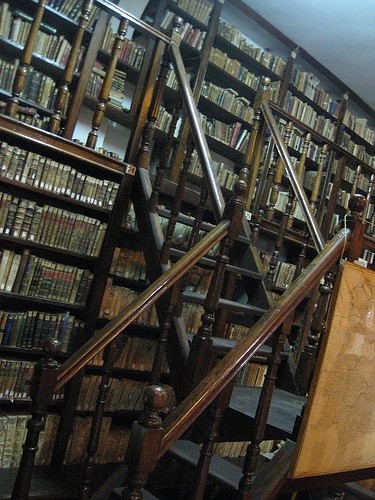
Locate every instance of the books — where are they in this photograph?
[0,0,375,500]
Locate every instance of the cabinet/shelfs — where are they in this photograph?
[0,0,375,500]
